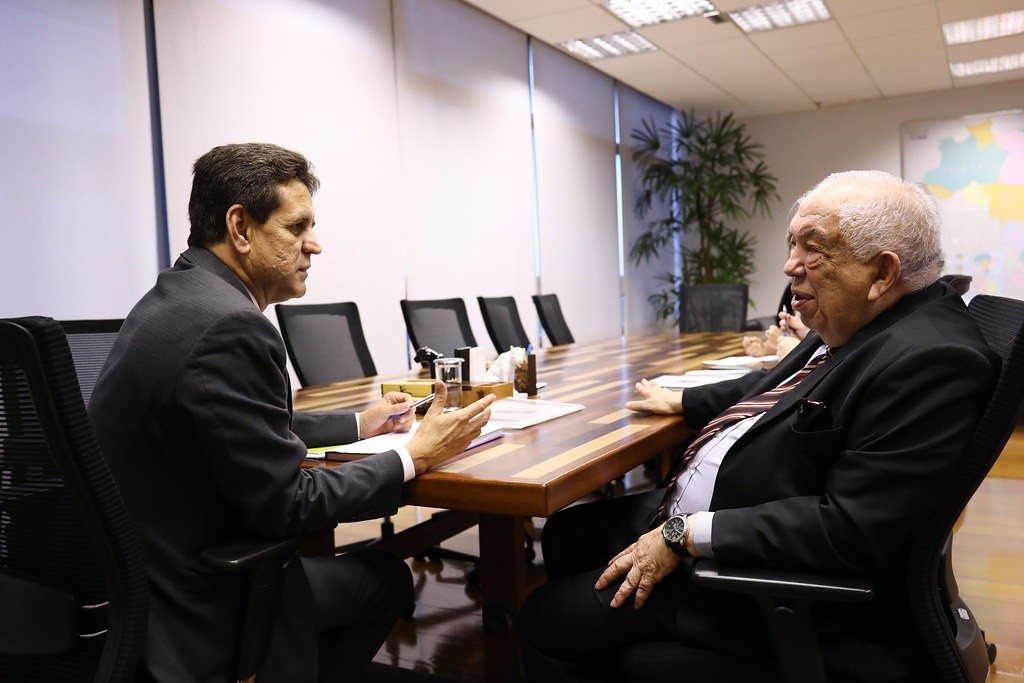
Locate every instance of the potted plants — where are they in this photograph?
[621,110,786,332]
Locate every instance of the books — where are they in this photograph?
[304,421,505,461]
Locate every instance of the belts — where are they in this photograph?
[647,510,693,574]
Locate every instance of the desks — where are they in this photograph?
[290,333,804,683]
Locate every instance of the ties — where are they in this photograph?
[646,346,837,531]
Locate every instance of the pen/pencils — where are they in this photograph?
[389,393,436,418]
[782,303,788,340]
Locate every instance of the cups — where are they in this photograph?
[433,357,462,414]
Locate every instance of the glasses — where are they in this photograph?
[797,397,834,432]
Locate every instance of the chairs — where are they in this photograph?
[944,275,973,295]
[275,301,379,389]
[532,293,573,345]
[478,296,531,354]
[0,314,404,683]
[59,319,126,410]
[400,297,478,370]
[586,295,1024,683]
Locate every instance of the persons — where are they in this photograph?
[69,143,497,683]
[517,170,1004,683]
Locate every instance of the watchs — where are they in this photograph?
[661,513,693,558]
